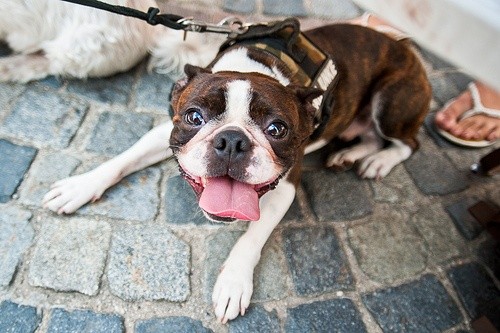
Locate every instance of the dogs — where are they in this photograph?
[40,22,435,324]
[0,0,205,84]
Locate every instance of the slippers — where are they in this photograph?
[434,79,500,147]
[361,11,408,41]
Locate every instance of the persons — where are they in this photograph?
[348,12,500,148]
